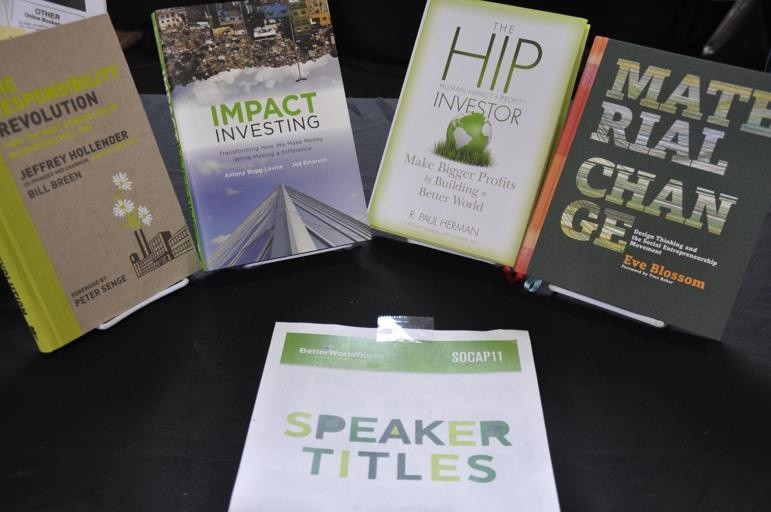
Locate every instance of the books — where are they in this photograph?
[365,0,591,267]
[0,0,106,41]
[147,0,377,273]
[512,35,770,346]
[230,323,566,512]
[0,11,204,356]
